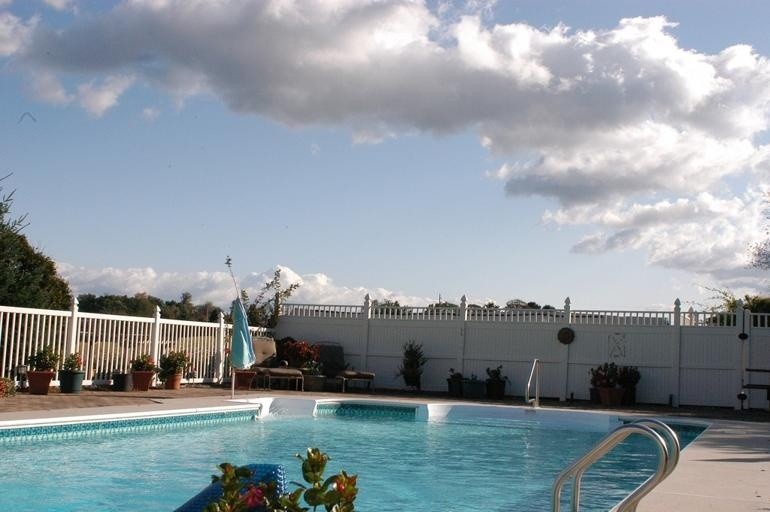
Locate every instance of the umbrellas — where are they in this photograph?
[229,297,256,398]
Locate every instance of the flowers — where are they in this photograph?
[64,351,85,368]
[132,354,156,371]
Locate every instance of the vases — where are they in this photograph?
[235,370,256,390]
[112,372,132,390]
[132,371,157,391]
[58,369,86,394]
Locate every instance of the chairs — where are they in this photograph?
[317,340,377,393]
[247,335,303,392]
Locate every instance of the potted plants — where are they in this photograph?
[586,362,640,408]
[394,340,429,391]
[302,341,326,393]
[22,345,59,394]
[159,349,192,390]
[446,363,511,400]
